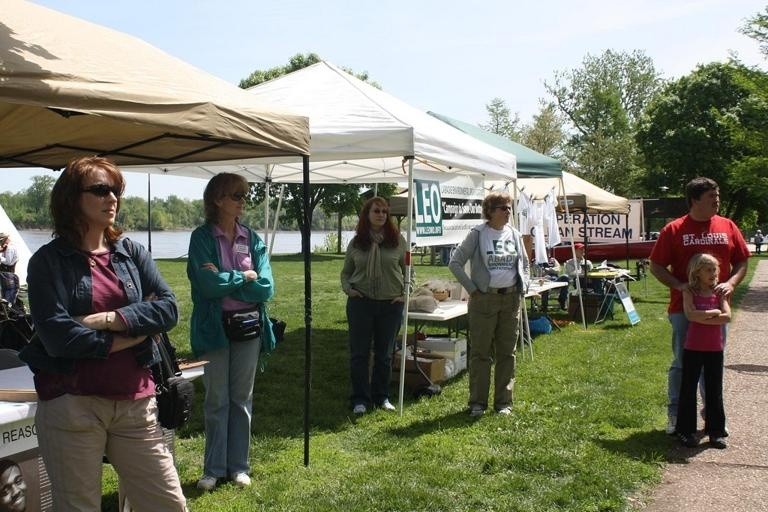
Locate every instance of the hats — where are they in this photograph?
[574,243,584,250]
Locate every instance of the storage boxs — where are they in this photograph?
[567,292,601,322]
[391,350,447,390]
[417,335,468,374]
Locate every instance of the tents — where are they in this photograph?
[0,204,32,284]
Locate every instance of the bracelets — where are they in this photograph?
[704,309,706,318]
[105,311,112,330]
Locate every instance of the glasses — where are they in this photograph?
[81,183,124,197]
[223,191,248,202]
[365,207,388,216]
[495,206,511,212]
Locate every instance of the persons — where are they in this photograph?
[17,155,187,512]
[449,192,530,417]
[649,177,752,437]
[340,196,414,415]
[531,245,568,311]
[187,173,276,491]
[676,253,731,448]
[0,460,27,512]
[754,230,763,254]
[0,232,19,301]
[564,243,592,288]
[0,233,19,273]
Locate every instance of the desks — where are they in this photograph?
[0,360,207,511]
[577,268,629,324]
[405,274,565,393]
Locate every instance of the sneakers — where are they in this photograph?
[353,404,366,414]
[231,469,252,487]
[381,400,395,411]
[197,473,217,490]
[667,409,729,449]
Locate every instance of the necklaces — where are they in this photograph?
[85,252,98,265]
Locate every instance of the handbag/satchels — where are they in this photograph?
[222,308,262,341]
[154,378,195,430]
